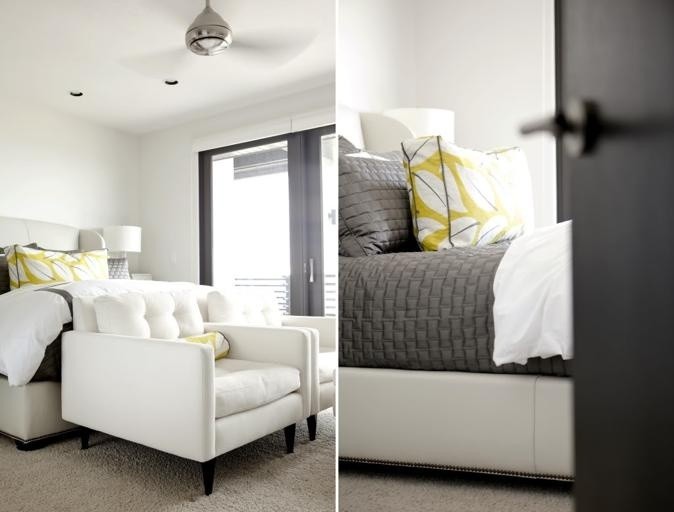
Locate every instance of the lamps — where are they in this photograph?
[107,226,143,259]
[184,1,229,57]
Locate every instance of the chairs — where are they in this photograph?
[59,288,335,496]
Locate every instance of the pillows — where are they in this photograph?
[5,244,108,291]
[337,135,525,258]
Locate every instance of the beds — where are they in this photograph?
[337,141,572,484]
[0,239,220,449]
